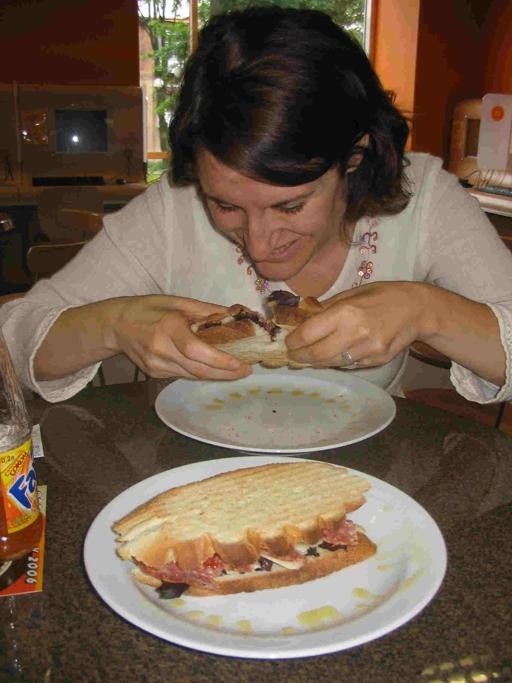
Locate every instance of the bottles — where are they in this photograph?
[0,327,44,563]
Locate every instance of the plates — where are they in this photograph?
[154,368,396,456]
[81,457,448,662]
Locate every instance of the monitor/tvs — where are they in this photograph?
[14,84,143,182]
[447,99,481,187]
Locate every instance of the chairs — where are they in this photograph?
[0,202,151,388]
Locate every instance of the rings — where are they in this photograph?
[342,351,355,363]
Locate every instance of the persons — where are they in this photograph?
[1,4,512,411]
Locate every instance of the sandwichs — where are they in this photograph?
[189,290,326,371]
[110,461,376,601]
